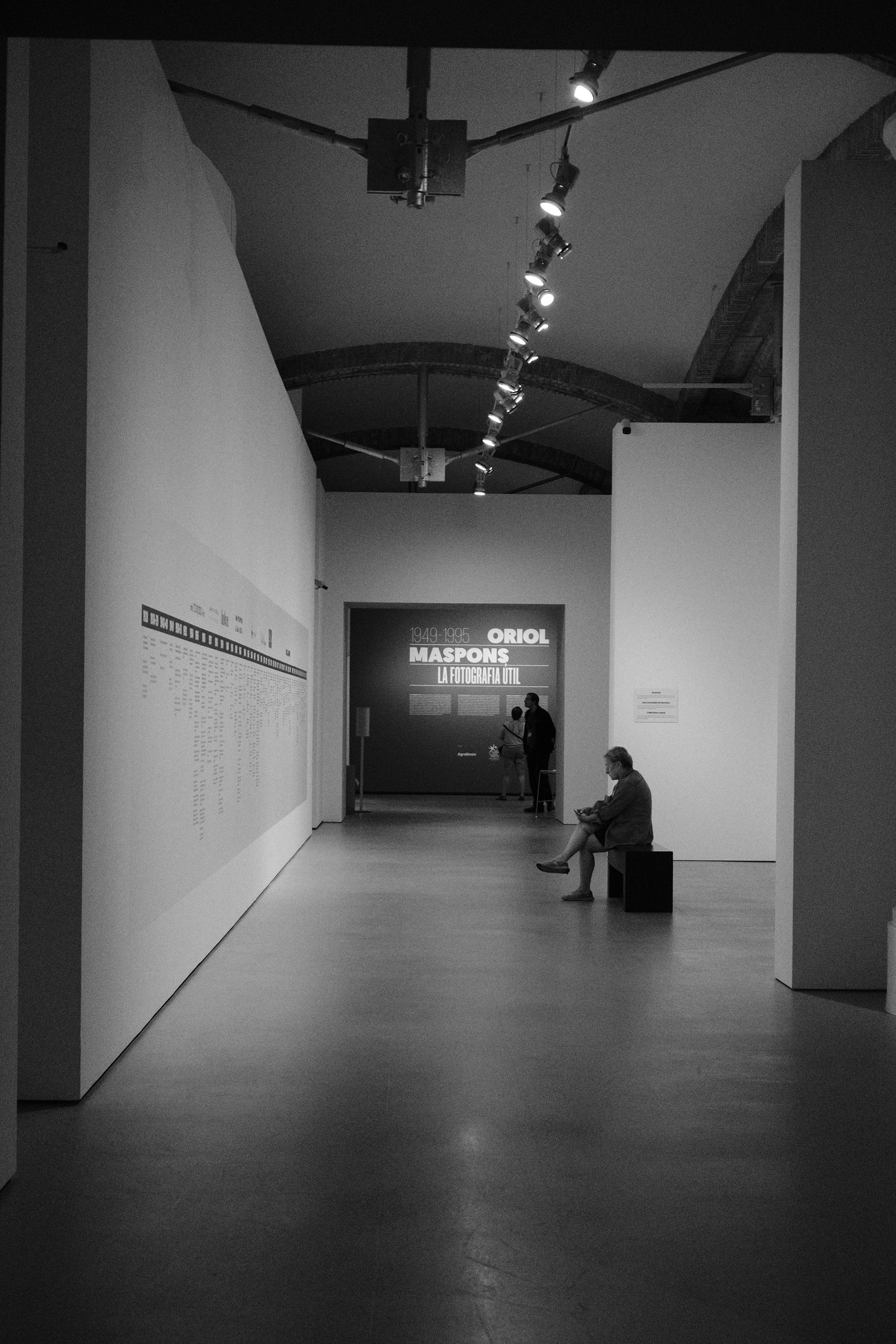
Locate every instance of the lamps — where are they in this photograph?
[356,706,371,813]
[473,47,619,496]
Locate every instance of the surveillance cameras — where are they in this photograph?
[621,419,632,434]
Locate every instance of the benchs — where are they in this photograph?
[607,840,674,913]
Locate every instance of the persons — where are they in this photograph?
[522,692,557,813]
[536,747,654,901]
[494,706,527,801]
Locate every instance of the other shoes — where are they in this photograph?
[524,801,545,812]
[496,795,507,800]
[518,797,524,800]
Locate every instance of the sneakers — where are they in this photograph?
[536,859,570,874]
[561,888,595,901]
